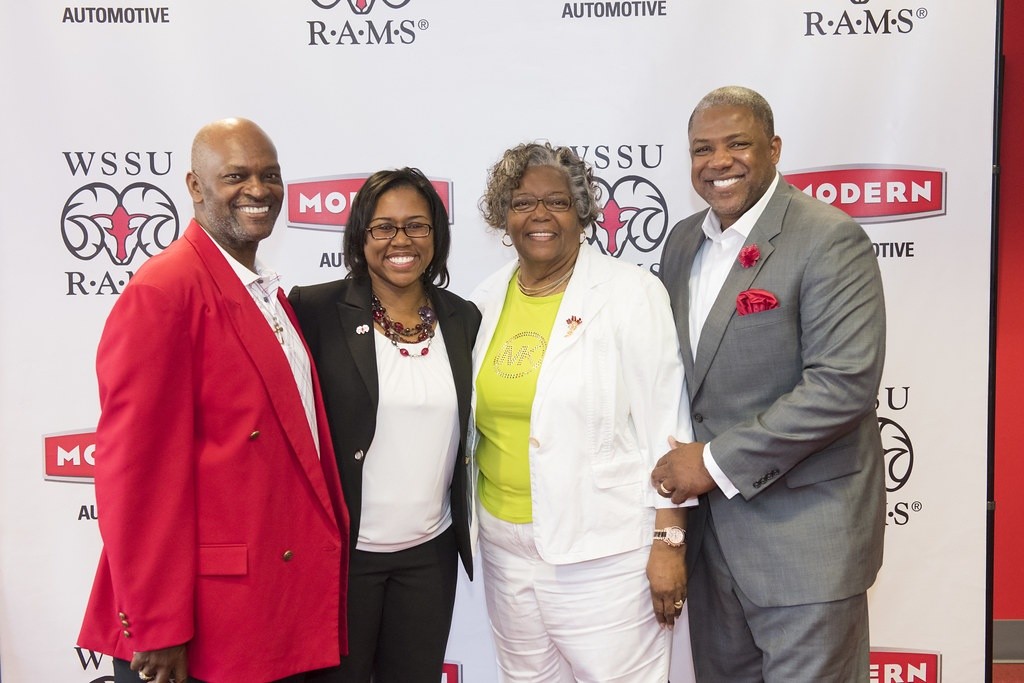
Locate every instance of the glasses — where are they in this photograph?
[366,223,434,240]
[510,191,576,213]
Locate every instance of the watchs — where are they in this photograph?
[653,526,687,548]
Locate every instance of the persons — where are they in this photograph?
[651,84,887,683]
[77,118,350,683]
[288,167,483,683]
[467,143,699,683]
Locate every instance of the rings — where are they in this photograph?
[139,671,152,681]
[674,600,683,609]
[660,484,672,494]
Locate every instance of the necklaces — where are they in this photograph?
[371,294,436,357]
[517,268,574,297]
[251,290,283,343]
